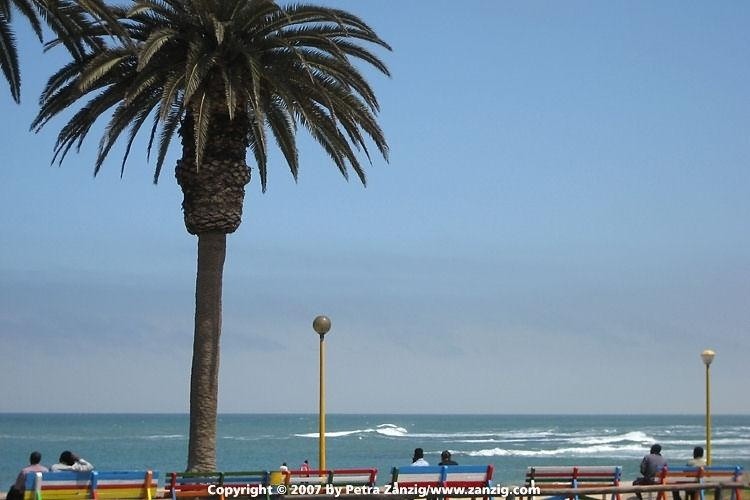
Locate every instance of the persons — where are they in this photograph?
[280,462,288,471]
[300,460,312,469]
[672,446,706,500]
[5,451,49,500]
[438,450,457,465]
[51,450,95,472]
[632,443,667,500]
[410,448,428,466]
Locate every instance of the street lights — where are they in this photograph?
[701,350,715,466]
[313,316,331,477]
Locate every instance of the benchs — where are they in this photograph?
[165,471,268,500]
[23,472,159,500]
[269,470,378,500]
[649,465,744,500]
[519,466,623,500]
[388,464,494,500]
